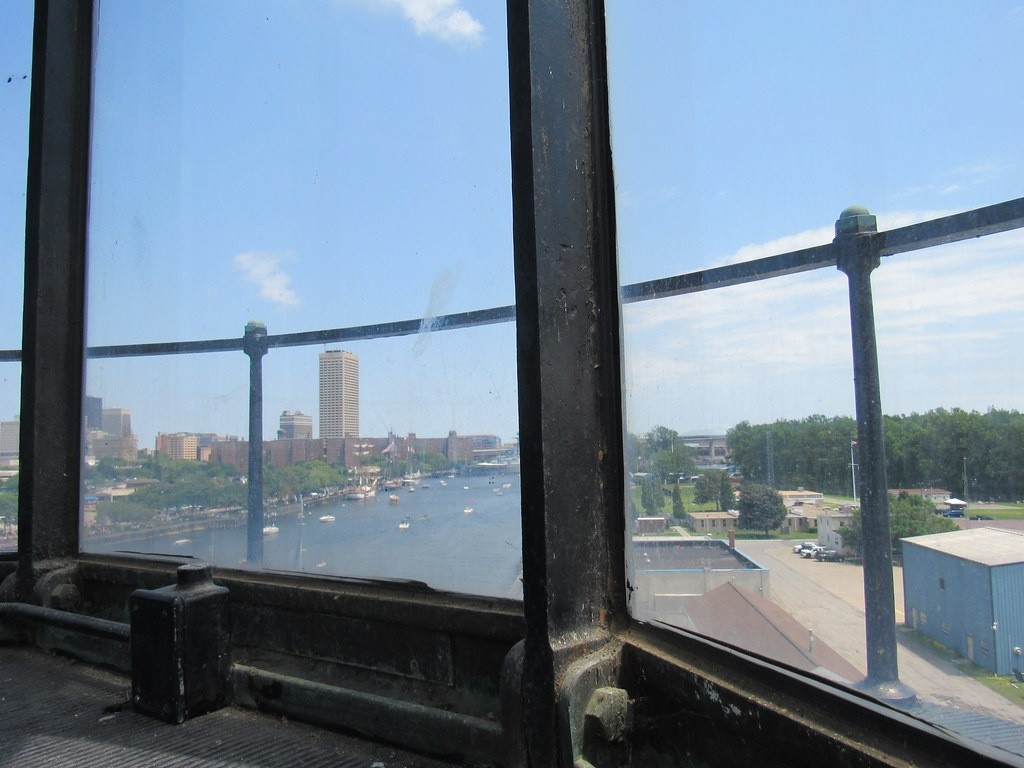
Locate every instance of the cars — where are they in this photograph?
[934,497,995,522]
[793,542,844,562]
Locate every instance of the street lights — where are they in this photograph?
[850,440,859,504]
